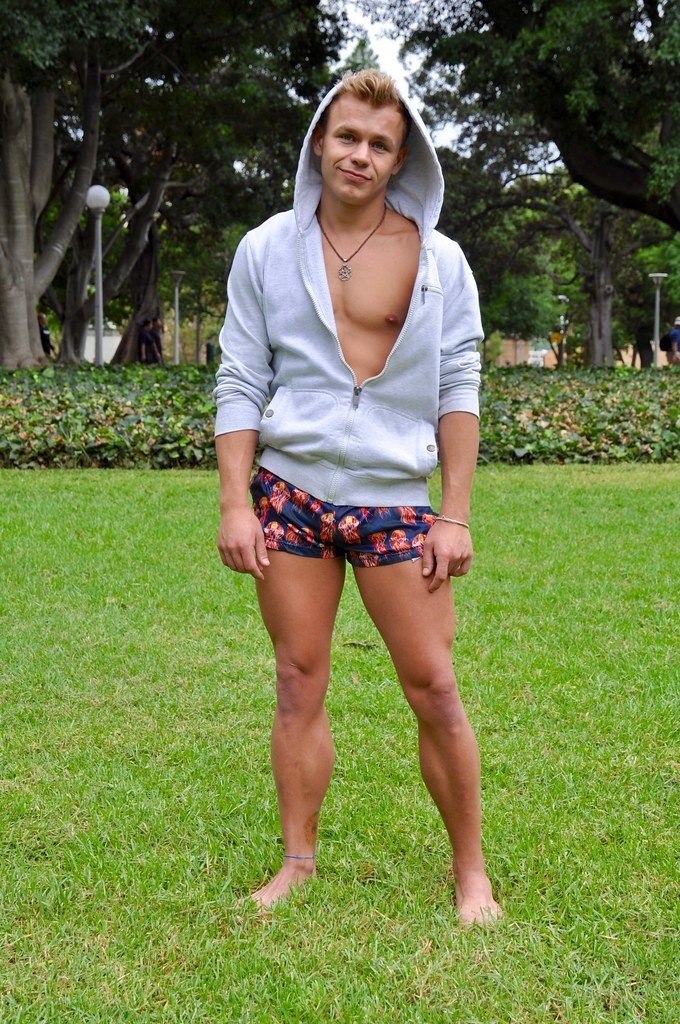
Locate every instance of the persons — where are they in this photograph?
[149,317,162,364]
[38,313,58,356]
[216,71,502,934]
[139,319,159,366]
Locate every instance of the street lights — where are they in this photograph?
[648,273,669,368]
[168,270,186,364]
[86,185,111,367]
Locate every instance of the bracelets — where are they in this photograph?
[436,516,469,528]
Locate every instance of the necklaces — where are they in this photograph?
[314,202,387,282]
